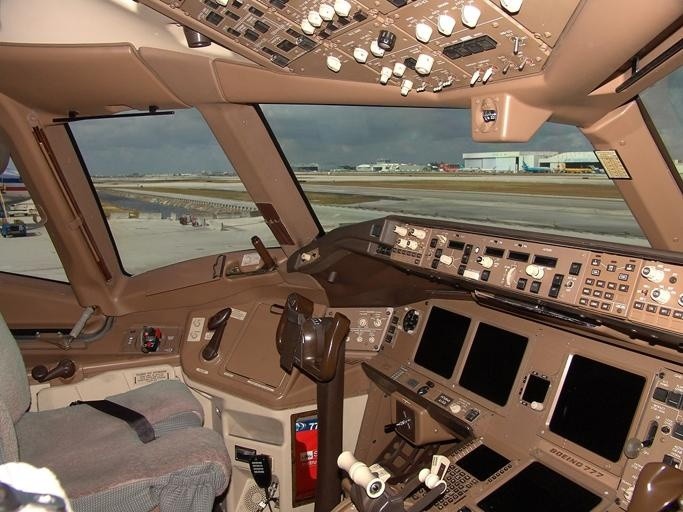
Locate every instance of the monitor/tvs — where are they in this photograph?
[402,301,476,391]
[536,345,657,477]
[465,447,618,512]
[450,317,537,418]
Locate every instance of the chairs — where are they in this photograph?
[0,313,231,512]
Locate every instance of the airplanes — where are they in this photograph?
[521,161,551,173]
[457,162,480,172]
[0,169,32,207]
